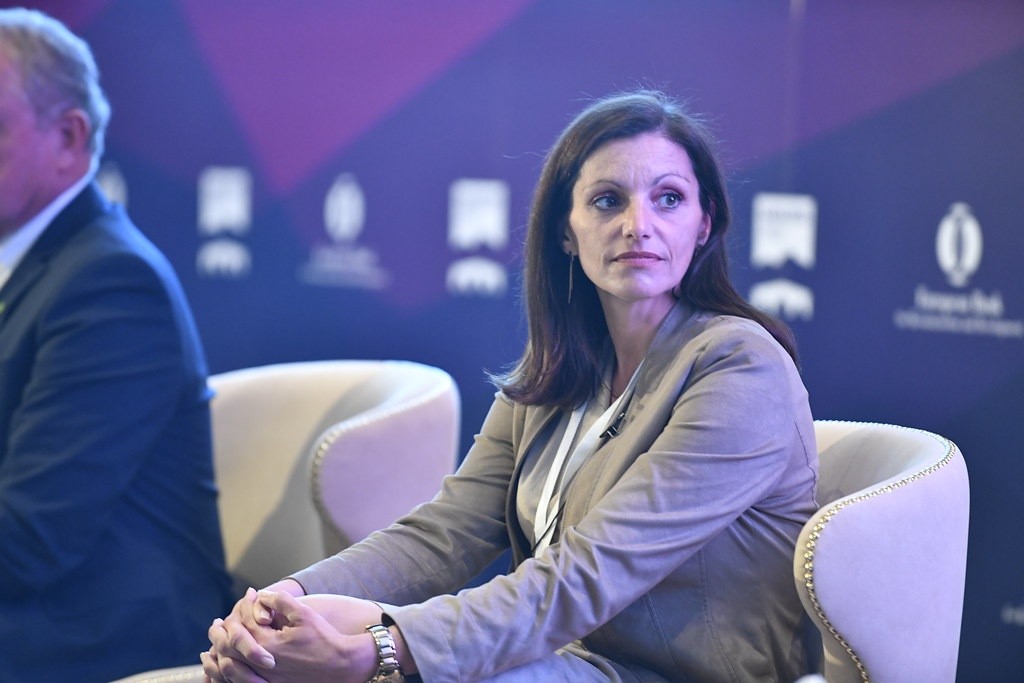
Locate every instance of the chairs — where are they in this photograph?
[107,357,464,683]
[803,416,971,683]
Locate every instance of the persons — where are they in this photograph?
[199,94,821,683]
[0,8,232,683]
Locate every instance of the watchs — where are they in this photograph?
[365,623,406,683]
